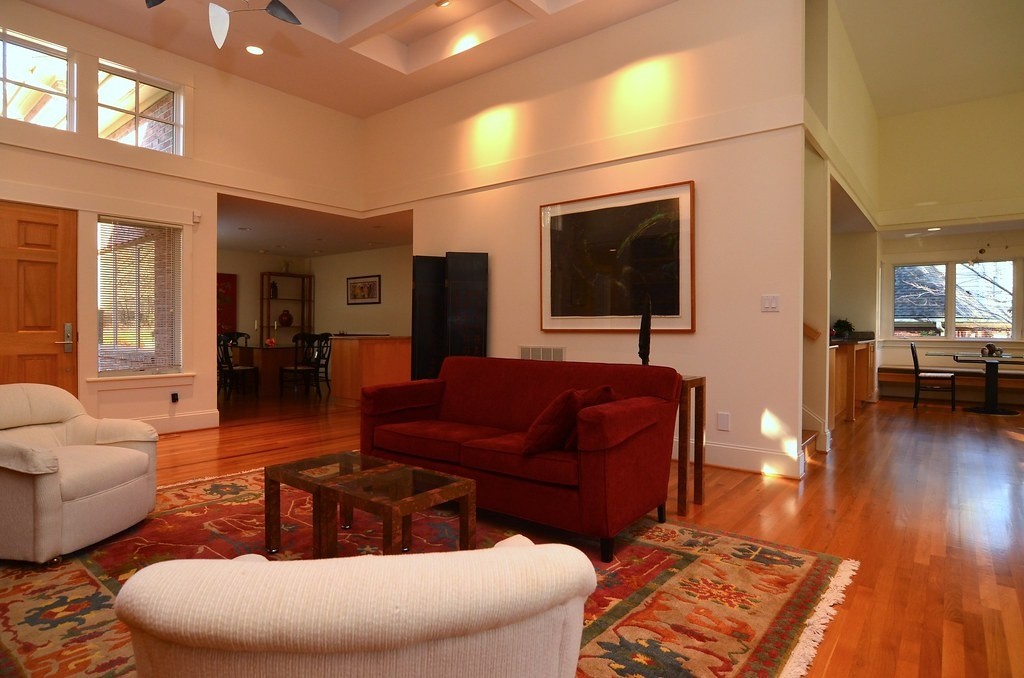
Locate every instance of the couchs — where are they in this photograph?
[0,381,160,571]
[114,533,599,677]
[359,354,681,562]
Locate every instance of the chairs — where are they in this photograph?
[908,342,956,411]
[218,332,259,402]
[280,332,332,399]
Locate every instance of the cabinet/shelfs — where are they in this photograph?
[259,271,318,345]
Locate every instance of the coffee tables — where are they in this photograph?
[265,448,480,561]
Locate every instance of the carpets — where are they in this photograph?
[0,448,861,677]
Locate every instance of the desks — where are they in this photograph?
[330,334,411,405]
[924,352,1024,416]
[232,342,303,404]
[831,336,876,423]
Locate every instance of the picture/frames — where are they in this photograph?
[540,181,696,335]
[346,274,382,305]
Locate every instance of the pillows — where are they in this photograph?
[522,387,591,457]
[563,383,623,453]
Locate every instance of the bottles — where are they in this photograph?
[985,343,995,356]
[995,347,1003,356]
[278,310,293,327]
[981,347,989,356]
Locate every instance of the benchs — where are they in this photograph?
[877,364,1024,389]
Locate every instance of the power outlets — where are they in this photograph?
[718,412,731,432]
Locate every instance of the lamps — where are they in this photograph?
[201,0,303,50]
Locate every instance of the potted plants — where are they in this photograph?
[833,317,854,339]
